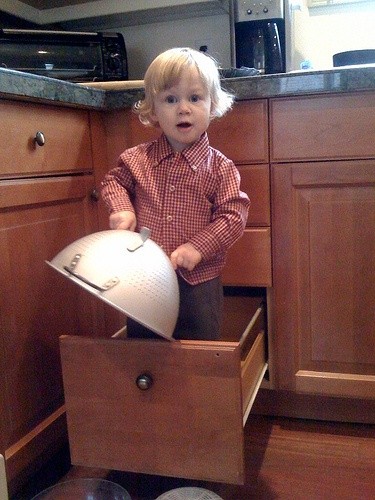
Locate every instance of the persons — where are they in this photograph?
[100,47,252,341]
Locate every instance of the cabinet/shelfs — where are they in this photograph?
[0,64,375,478]
